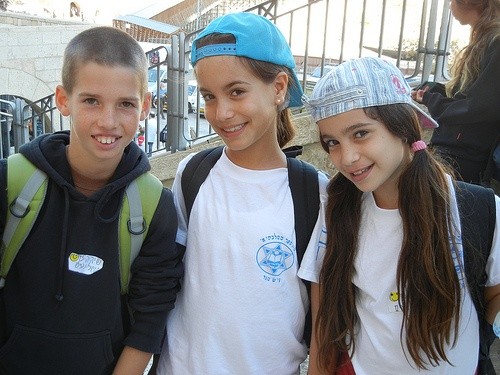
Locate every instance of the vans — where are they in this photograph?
[149,69,204,117]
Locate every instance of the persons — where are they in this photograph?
[141,111,158,152]
[146,12,329,375]
[297,56,500,375]
[0,26,184,375]
[410,1,499,199]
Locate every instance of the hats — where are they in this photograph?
[310,57,438,129]
[191,12,303,107]
